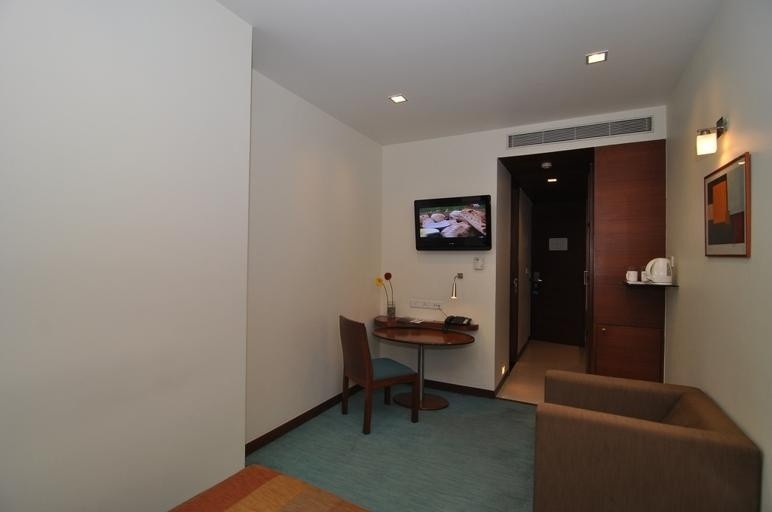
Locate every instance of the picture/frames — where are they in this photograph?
[703,150,751,258]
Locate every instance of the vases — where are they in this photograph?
[387,301,395,320]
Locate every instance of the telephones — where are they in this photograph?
[445,315,472,325]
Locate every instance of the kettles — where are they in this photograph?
[645,257,673,285]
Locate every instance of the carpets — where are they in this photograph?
[169,463,372,512]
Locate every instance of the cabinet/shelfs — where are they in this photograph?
[583,140,666,382]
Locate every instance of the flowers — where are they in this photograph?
[375,272,393,307]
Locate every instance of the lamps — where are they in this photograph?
[450,272,463,299]
[696,116,727,156]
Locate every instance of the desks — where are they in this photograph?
[372,327,474,410]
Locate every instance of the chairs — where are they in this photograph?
[339,315,420,434]
[533,369,762,512]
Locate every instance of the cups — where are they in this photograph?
[641,271,650,283]
[625,271,638,283]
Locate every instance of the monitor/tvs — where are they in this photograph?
[414,194,492,251]
